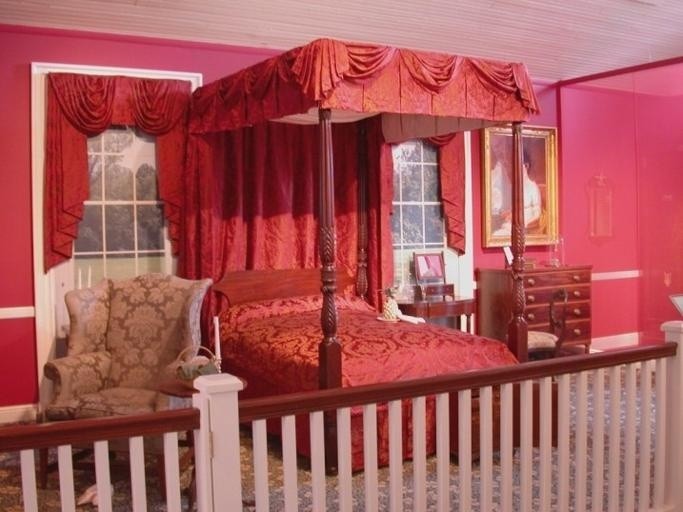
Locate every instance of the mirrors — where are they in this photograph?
[412,252,447,283]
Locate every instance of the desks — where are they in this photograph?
[395,285,476,333]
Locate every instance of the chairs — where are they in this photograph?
[43,271,212,512]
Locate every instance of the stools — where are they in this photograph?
[505,330,559,363]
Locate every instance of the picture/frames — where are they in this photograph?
[481,123,560,248]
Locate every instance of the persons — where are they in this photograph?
[491,147,542,236]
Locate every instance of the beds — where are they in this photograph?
[191,39,538,475]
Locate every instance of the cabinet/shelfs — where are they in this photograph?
[477,266,593,354]
